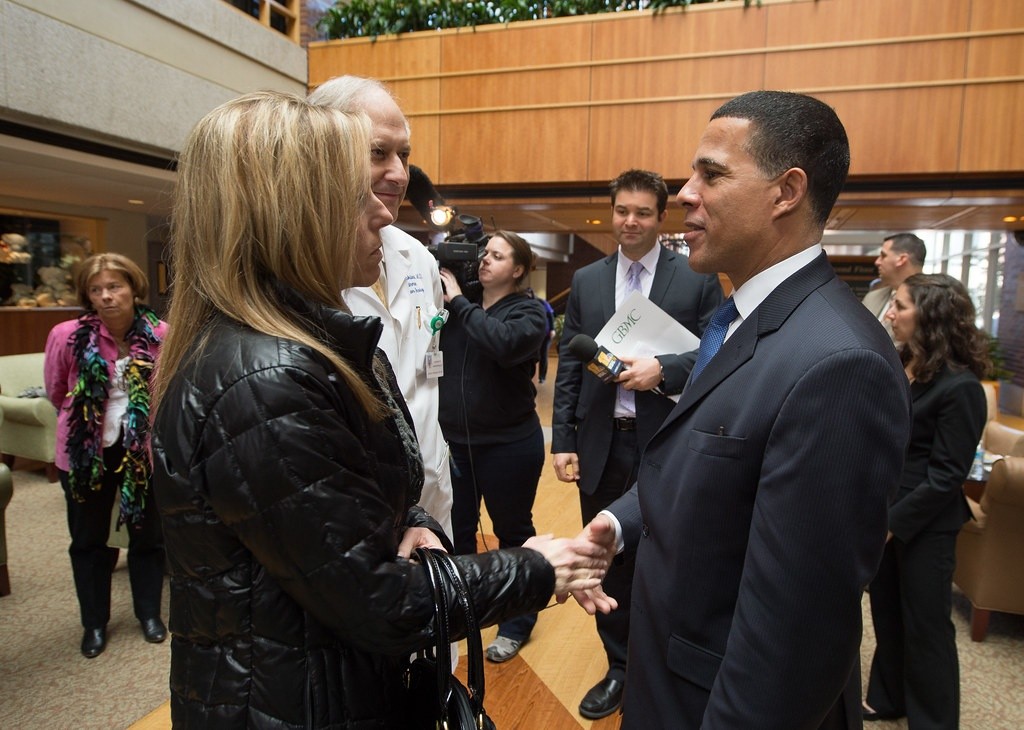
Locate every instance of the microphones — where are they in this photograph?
[566,333,628,385]
[405,165,455,232]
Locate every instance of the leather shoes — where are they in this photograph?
[80,623,106,658]
[578,673,625,718]
[138,614,166,643]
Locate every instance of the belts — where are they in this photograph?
[614,417,636,430]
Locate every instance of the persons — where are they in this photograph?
[149,94,608,730]
[860,233,927,357]
[549,172,726,719]
[856,236,997,730]
[526,287,556,384]
[43,254,171,659]
[312,74,461,677]
[577,92,910,730]
[439,231,552,664]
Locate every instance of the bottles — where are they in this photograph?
[970,440,983,479]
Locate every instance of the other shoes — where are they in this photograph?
[485,636,524,662]
[861,699,885,719]
[538,379,544,383]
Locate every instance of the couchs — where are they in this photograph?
[944,380,1023,641]
[0,352,62,476]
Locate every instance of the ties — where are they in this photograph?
[690,297,740,386]
[618,261,644,414]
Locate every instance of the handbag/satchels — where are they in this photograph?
[397,547,495,730]
